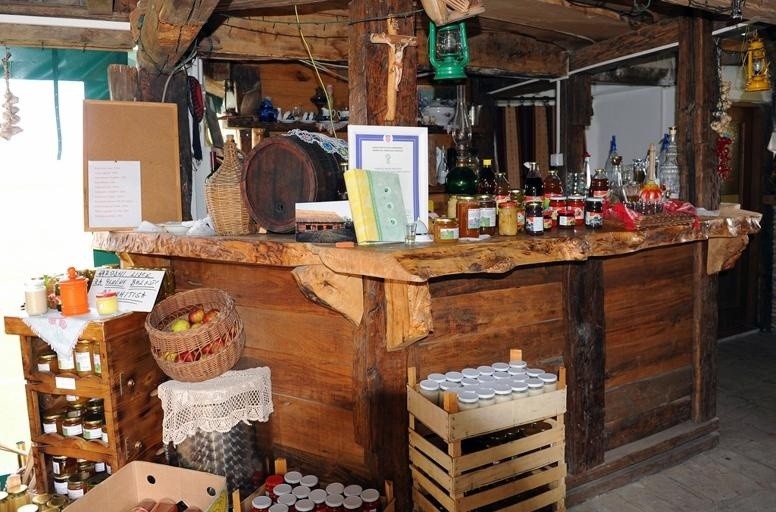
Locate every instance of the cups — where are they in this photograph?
[404,223,419,244]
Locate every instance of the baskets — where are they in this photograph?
[203,141,261,236]
[144,287,244,380]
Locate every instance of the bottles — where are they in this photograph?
[124,496,203,512]
[446,156,477,197]
[590,168,609,198]
[57,349,76,377]
[478,159,495,194]
[74,339,94,376]
[24,280,48,316]
[545,170,562,200]
[92,342,102,377]
[523,162,544,204]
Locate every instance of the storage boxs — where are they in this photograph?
[232,455,397,512]
[59,460,230,512]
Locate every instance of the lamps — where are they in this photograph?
[743,33,771,94]
[429,17,470,82]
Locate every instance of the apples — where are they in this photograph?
[156,304,236,374]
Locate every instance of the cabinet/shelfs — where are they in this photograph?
[406,347,568,512]
[3,311,170,498]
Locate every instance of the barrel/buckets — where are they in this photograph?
[239,134,345,235]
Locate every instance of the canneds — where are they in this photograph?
[433,218,459,245]
[548,197,567,208]
[456,194,481,239]
[558,209,577,230]
[251,473,381,511]
[420,360,560,412]
[584,197,604,230]
[482,422,552,466]
[37,353,59,374]
[567,195,584,227]
[47,459,110,501]
[543,209,554,231]
[1,482,67,512]
[41,395,108,445]
[526,200,545,235]
[477,194,495,237]
[497,201,517,238]
